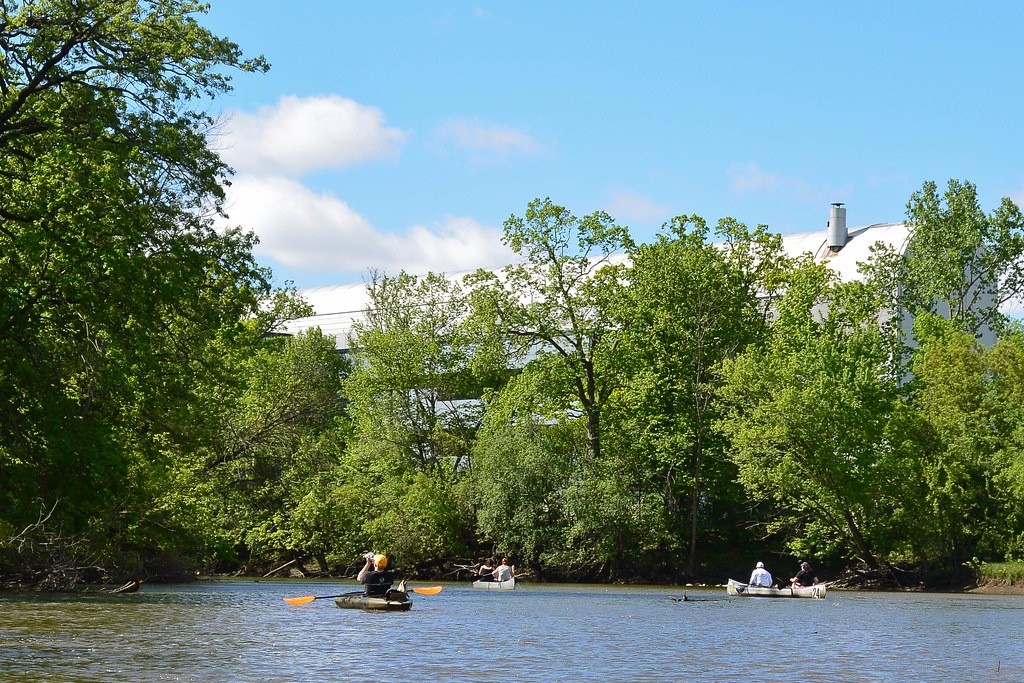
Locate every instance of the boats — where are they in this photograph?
[727,578,827,598]
[334,589,414,612]
[470,577,517,591]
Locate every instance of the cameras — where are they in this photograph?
[363,553,375,562]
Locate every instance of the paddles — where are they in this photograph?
[282,586,443,607]
[514,573,531,577]
[736,584,754,594]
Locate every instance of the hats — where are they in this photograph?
[756,562,764,568]
[374,554,387,568]
[800,562,808,566]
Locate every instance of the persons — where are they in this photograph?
[478,559,495,582]
[356,554,394,598]
[749,561,773,588]
[789,561,818,589]
[491,558,512,582]
